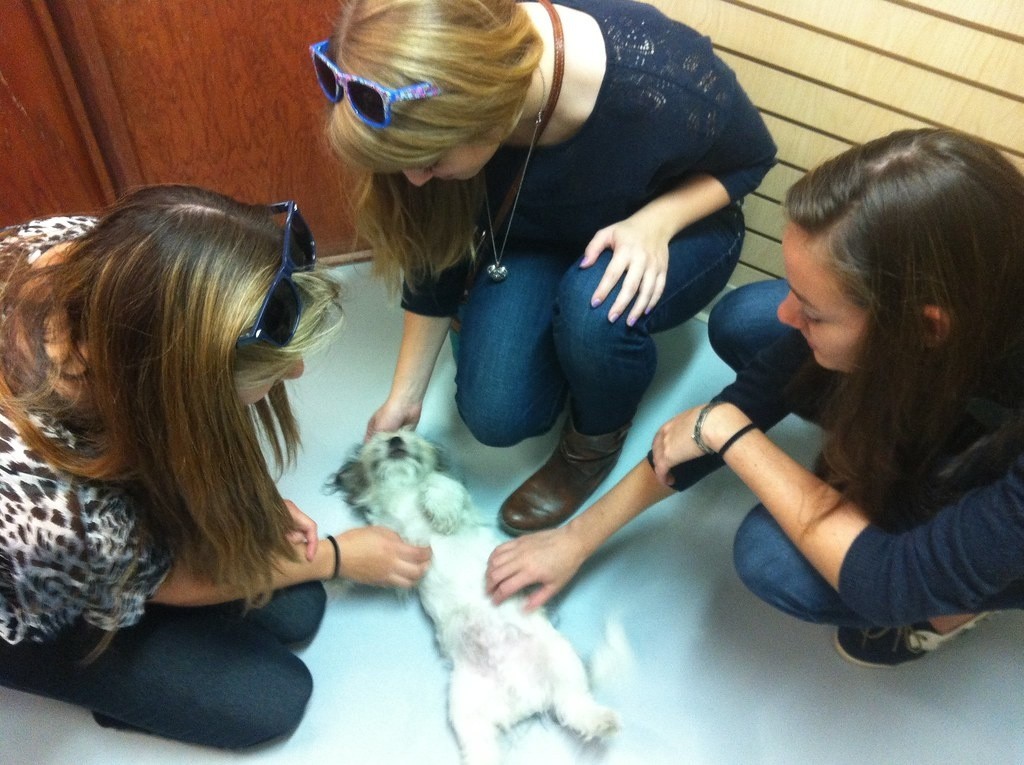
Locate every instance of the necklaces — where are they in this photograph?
[483,62,548,282]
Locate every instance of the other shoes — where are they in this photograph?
[833,611,992,669]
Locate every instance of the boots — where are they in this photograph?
[497,413,634,538]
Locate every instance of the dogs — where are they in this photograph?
[320,420,622,765]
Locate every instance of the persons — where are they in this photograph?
[324,0,780,537]
[488,127,1023,669]
[0,185,433,753]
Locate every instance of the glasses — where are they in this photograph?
[309,39,442,128]
[230,199,316,350]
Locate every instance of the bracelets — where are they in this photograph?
[327,534,341,582]
[693,400,727,456]
[718,422,758,459]
